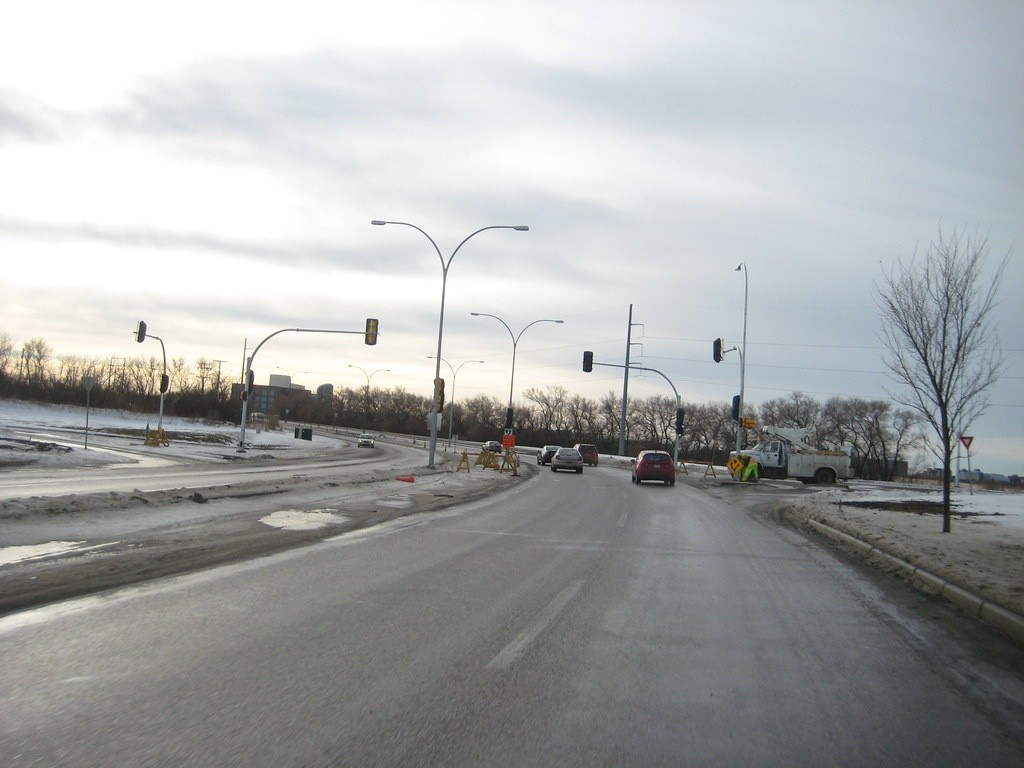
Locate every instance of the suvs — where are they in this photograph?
[573,444,599,467]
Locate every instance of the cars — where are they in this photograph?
[536,445,562,466]
[631,449,676,486]
[550,447,584,473]
[357,434,375,448]
[482,440,502,454]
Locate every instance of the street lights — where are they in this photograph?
[427,357,483,448]
[470,312,563,434]
[735,263,748,453]
[371,220,528,470]
[277,366,308,394]
[349,365,390,434]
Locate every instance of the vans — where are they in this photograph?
[252,412,265,424]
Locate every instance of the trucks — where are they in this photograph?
[729,435,850,486]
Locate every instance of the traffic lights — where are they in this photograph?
[712,337,725,364]
[240,391,248,401]
